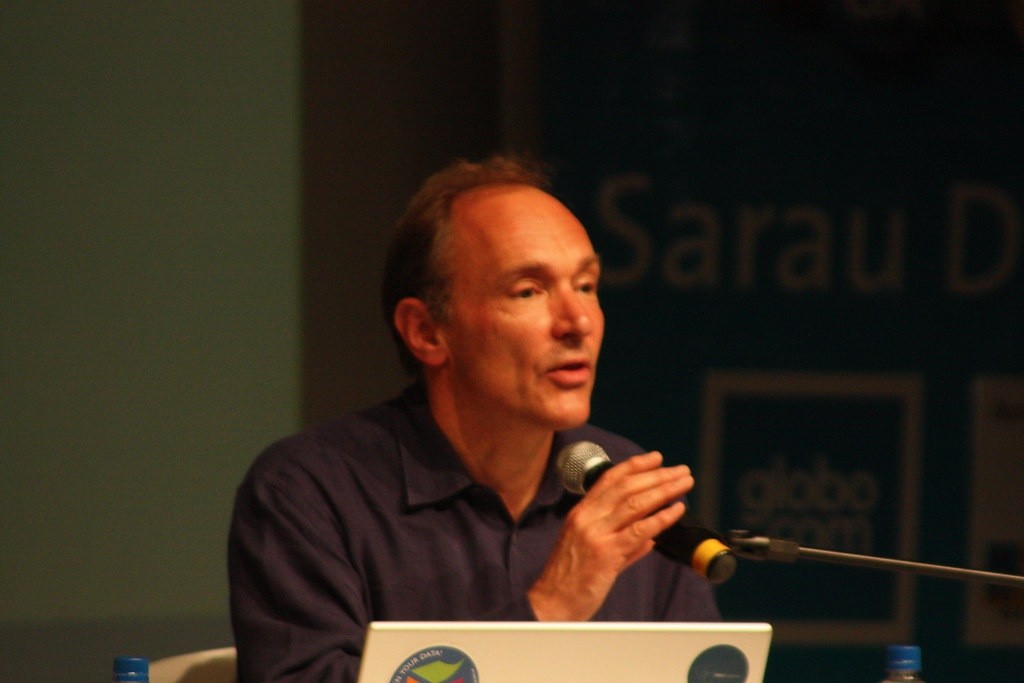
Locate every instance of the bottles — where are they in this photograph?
[113,656,149,683]
[880,645,925,683]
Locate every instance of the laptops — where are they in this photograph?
[358,622,773,683]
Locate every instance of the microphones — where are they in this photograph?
[554,441,737,584]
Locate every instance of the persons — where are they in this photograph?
[226,155,724,683]
